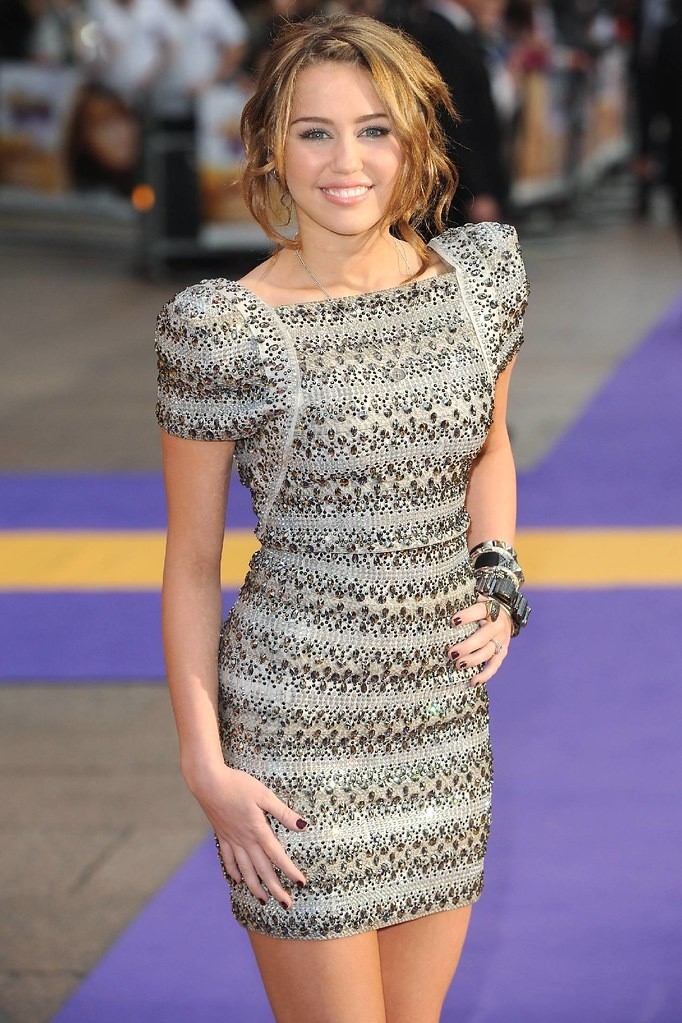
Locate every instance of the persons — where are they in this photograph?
[152,17,531,1023]
[0,0,682,269]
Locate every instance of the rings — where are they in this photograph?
[479,600,500,622]
[491,639,502,656]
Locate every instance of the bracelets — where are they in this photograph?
[469,540,531,637]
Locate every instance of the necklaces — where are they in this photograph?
[293,232,422,382]
